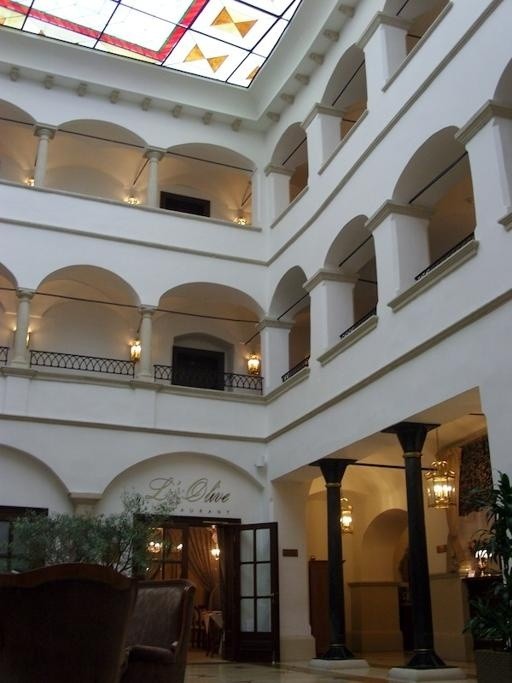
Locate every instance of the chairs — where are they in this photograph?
[191,605,224,658]
[116,580,197,683]
[0,563,138,683]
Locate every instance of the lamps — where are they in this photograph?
[128,194,137,204]
[131,340,141,361]
[248,352,261,375]
[27,176,34,186]
[475,550,492,577]
[238,216,246,226]
[424,430,457,508]
[339,497,354,534]
